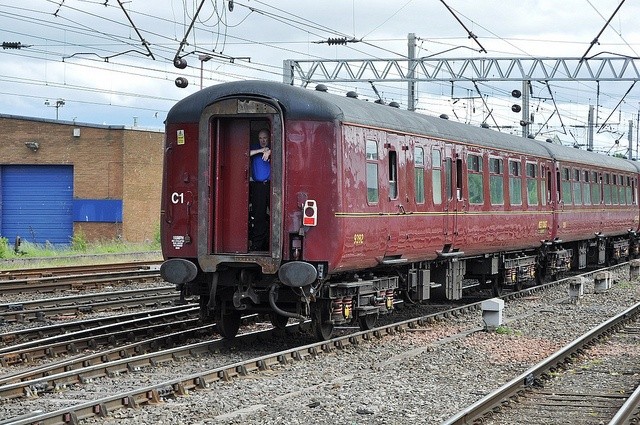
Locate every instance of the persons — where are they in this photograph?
[250,128,271,183]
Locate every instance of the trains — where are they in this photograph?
[160,80,640,340]
